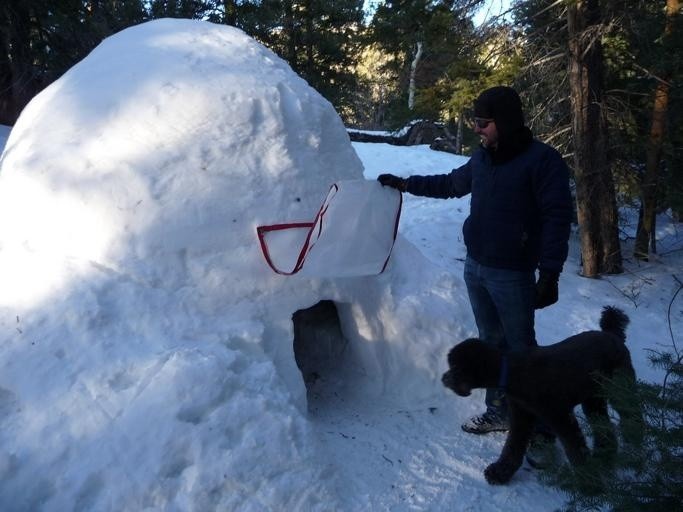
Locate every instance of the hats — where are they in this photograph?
[471,85,525,149]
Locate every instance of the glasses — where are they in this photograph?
[468,117,496,130]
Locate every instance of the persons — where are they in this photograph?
[378,86,572,470]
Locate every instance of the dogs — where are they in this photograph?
[441,304,647,498]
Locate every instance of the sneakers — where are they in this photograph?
[524,430,559,471]
[459,410,511,437]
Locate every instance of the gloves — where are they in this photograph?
[531,267,560,310]
[376,172,408,193]
